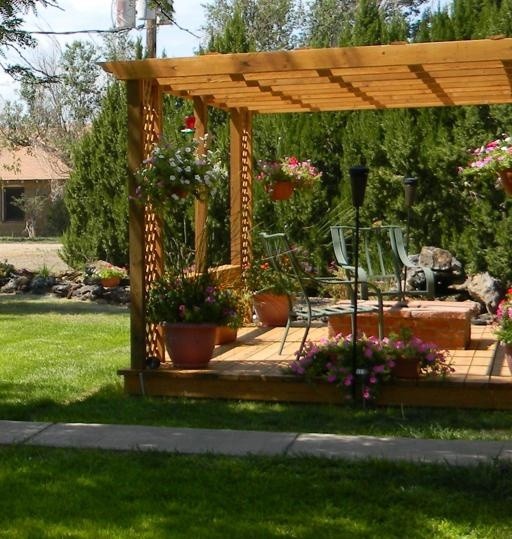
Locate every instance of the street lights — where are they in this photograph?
[344,161,370,421]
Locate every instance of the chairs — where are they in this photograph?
[261,222,437,363]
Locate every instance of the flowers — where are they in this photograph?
[94,260,127,279]
[457,138,512,185]
[255,154,322,192]
[146,249,245,325]
[293,329,451,400]
[490,287,512,344]
[128,136,228,206]
[246,253,303,289]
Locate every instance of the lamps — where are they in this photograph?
[348,166,370,406]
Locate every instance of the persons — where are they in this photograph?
[31,215,36,236]
[25,217,33,238]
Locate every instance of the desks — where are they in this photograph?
[328,300,479,350]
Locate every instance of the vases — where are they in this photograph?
[172,187,189,198]
[268,180,293,200]
[252,290,295,327]
[492,167,512,197]
[101,276,120,287]
[500,339,512,371]
[164,327,217,370]
[211,322,237,346]
